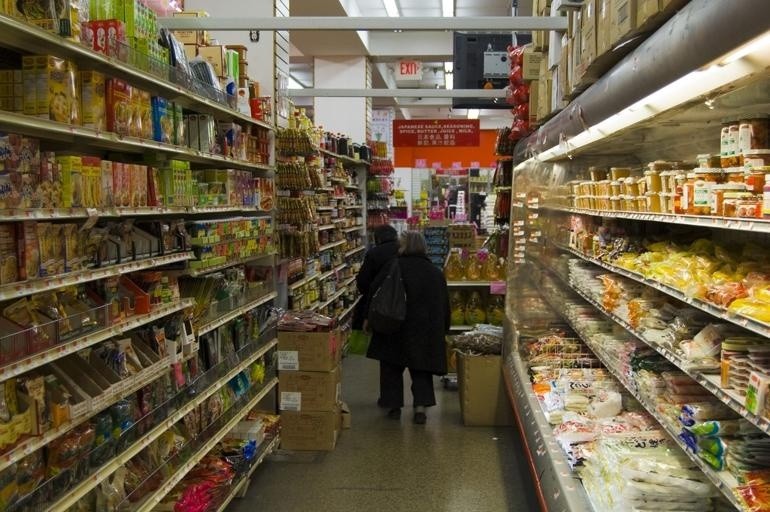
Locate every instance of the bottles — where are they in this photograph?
[286,106,373,325]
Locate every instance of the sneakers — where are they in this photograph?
[375,393,429,425]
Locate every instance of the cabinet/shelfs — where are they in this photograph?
[504,27,770,512]
[0,15,408,512]
[440,279,506,389]
[412,167,490,224]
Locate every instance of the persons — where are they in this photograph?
[360,229,453,424]
[354,224,403,408]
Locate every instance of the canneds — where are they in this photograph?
[673,119,770,218]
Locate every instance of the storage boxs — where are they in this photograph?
[456,351,515,427]
[522,0,690,129]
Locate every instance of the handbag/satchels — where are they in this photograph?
[368,273,410,320]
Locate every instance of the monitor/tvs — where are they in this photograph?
[452,30,531,109]
